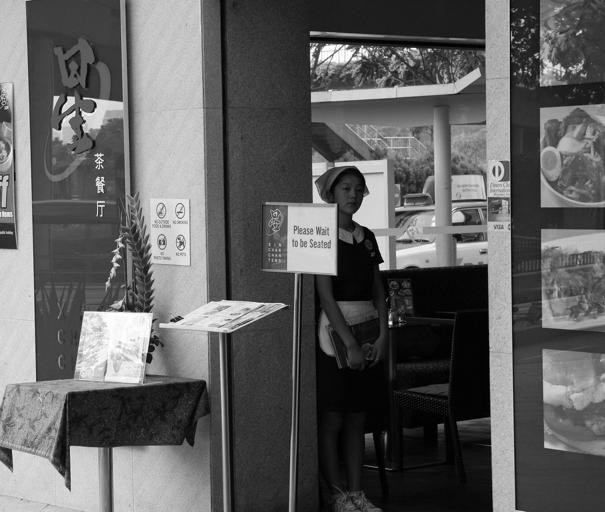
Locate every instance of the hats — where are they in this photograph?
[315,166,369,202]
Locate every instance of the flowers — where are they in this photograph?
[97,192,167,364]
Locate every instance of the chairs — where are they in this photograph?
[391,309,488,478]
[371,311,451,469]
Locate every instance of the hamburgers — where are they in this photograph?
[543,351,605,456]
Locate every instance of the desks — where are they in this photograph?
[0,373,211,511]
[363,315,455,471]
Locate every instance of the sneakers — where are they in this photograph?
[331,491,381,511]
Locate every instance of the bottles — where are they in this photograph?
[387,278,415,328]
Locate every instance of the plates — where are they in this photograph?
[540,233,605,330]
[540,114,605,208]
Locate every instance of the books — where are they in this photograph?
[325,310,390,371]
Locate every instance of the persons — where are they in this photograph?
[314,166,390,512]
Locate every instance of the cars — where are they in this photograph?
[32,196,126,282]
[395,169,491,270]
